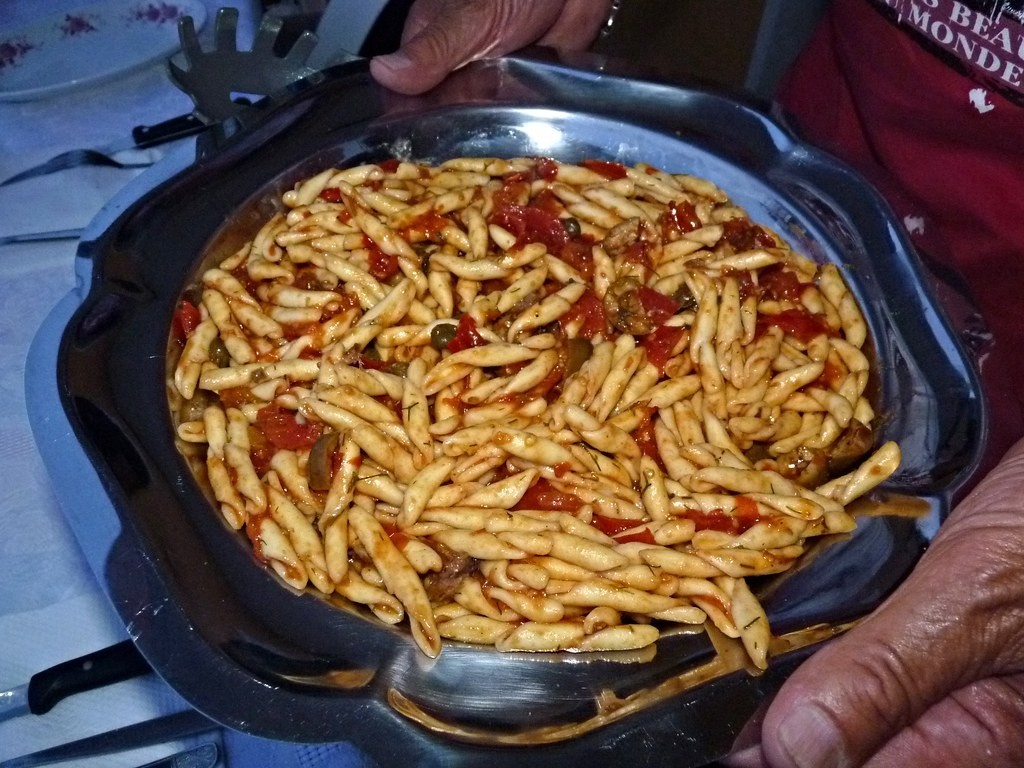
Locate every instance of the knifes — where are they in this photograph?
[0,97,253,187]
[0,637,156,724]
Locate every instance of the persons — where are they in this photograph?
[368,0,1024,768]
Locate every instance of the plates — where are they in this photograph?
[22,59,987,768]
[0,0,208,103]
